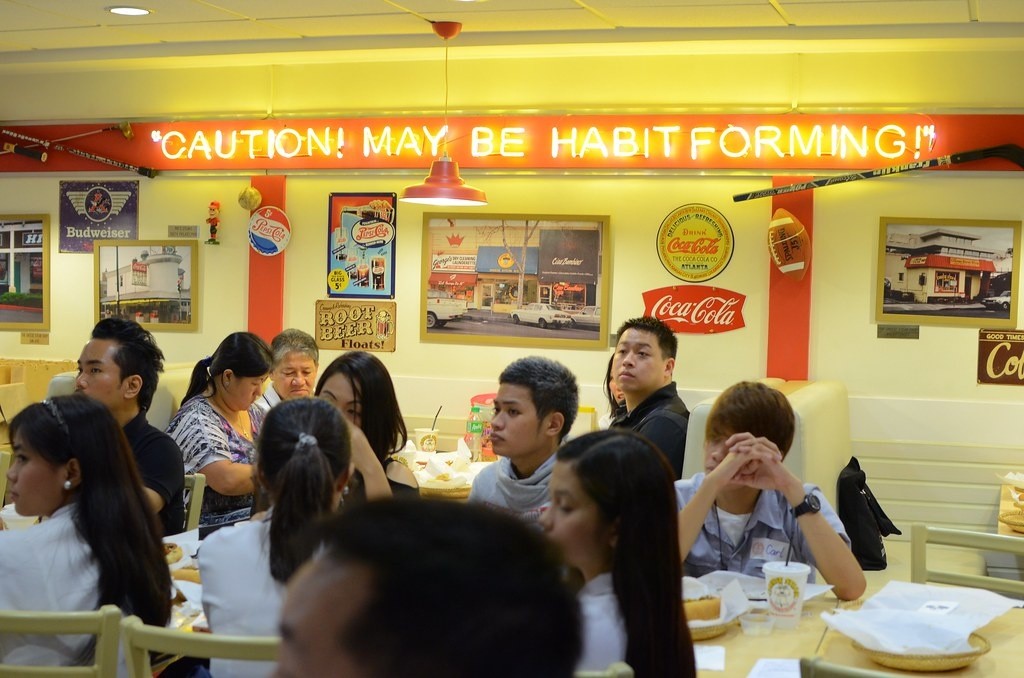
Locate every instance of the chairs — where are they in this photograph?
[0,602,121,678]
[184,473,205,531]
[910,523,1024,601]
[118,614,286,678]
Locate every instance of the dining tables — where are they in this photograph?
[389,453,504,507]
[158,524,213,634]
[682,583,1024,678]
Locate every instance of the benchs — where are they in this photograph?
[48,360,199,434]
[681,374,987,591]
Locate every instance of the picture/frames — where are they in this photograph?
[421,213,613,352]
[874,216,1024,330]
[0,214,51,332]
[95,239,200,333]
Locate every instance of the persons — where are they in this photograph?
[602,352,626,420]
[246,326,320,438]
[671,379,868,602]
[466,354,580,533]
[206,200,221,241]
[607,314,690,483]
[160,329,270,543]
[535,427,698,678]
[258,493,588,678]
[313,350,421,513]
[195,394,357,678]
[71,316,186,539]
[0,393,175,678]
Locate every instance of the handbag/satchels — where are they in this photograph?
[838,458,902,572]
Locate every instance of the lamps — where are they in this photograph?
[397,20,488,207]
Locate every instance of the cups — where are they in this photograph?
[761,559,810,630]
[415,428,437,455]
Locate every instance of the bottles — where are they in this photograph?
[466,405,482,461]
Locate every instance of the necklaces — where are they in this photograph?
[208,389,249,437]
[714,489,765,571]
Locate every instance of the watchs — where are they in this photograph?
[788,492,820,519]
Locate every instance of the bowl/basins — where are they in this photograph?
[738,613,775,637]
